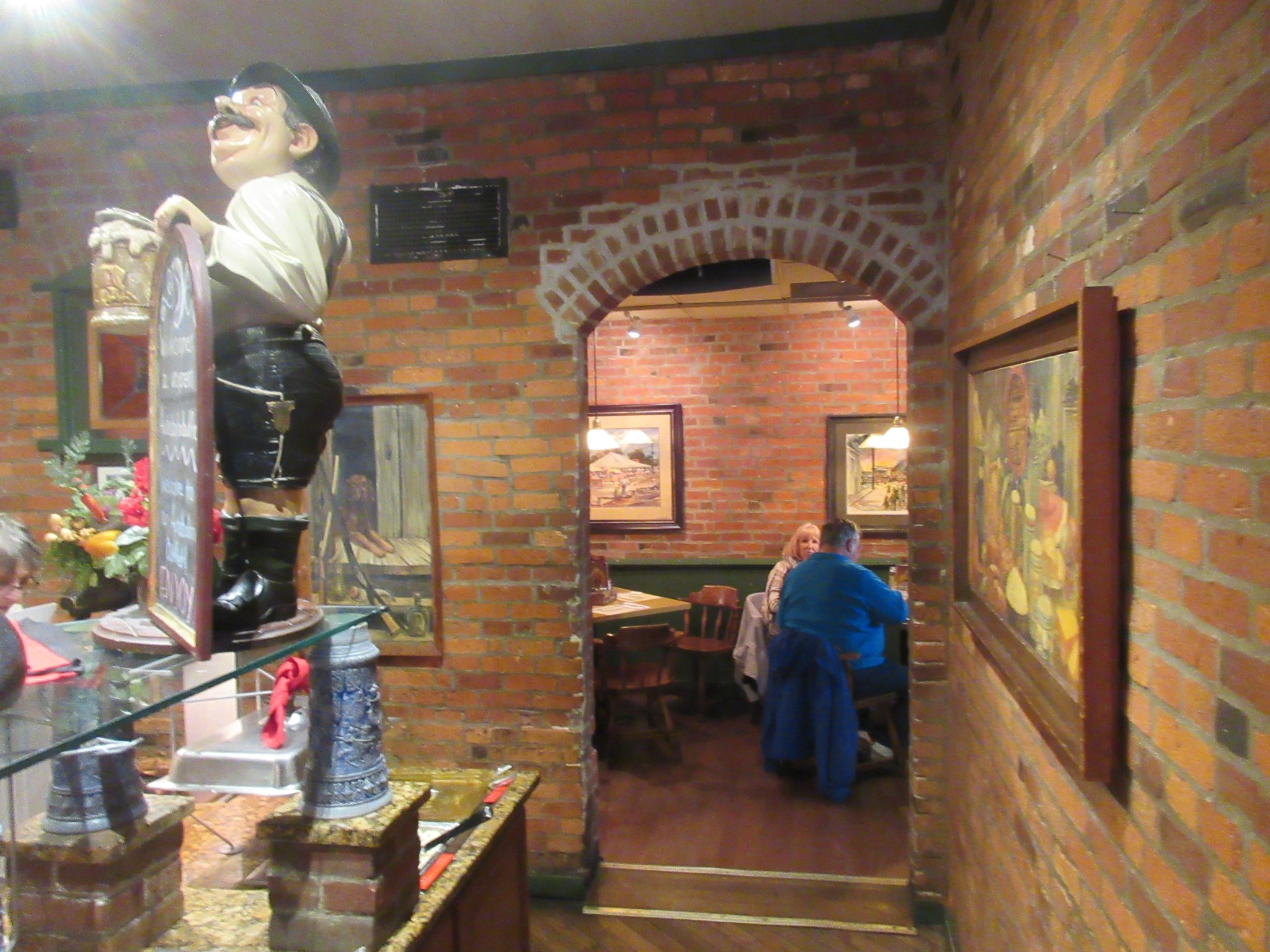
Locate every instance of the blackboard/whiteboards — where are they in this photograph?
[148,222,214,661]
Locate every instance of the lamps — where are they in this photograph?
[584,321,622,454]
[835,298,864,330]
[623,309,643,341]
[618,427,656,446]
[858,431,883,452]
[872,314,911,452]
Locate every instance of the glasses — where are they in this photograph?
[0,576,40,599]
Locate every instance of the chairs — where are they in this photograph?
[592,562,909,783]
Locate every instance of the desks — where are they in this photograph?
[592,583,693,629]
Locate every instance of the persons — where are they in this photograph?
[761,524,821,642]
[0,513,43,627]
[156,59,354,638]
[777,518,910,767]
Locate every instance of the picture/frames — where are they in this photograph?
[288,390,449,663]
[943,283,1132,787]
[586,402,689,536]
[82,302,156,436]
[823,411,912,542]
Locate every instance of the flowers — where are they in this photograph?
[42,433,226,594]
